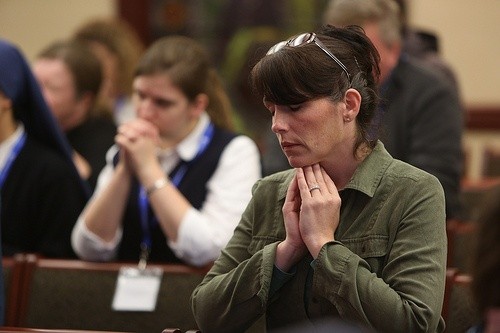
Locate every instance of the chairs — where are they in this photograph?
[0,178,497,333]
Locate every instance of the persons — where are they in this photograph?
[467,186,500,333]
[191,24,447,332]
[0,0,477,275]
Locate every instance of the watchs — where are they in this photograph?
[147,177,171,197]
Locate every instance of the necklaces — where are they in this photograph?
[1,134,26,181]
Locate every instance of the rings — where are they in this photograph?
[309,185,321,192]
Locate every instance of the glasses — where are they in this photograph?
[266,31,354,83]
